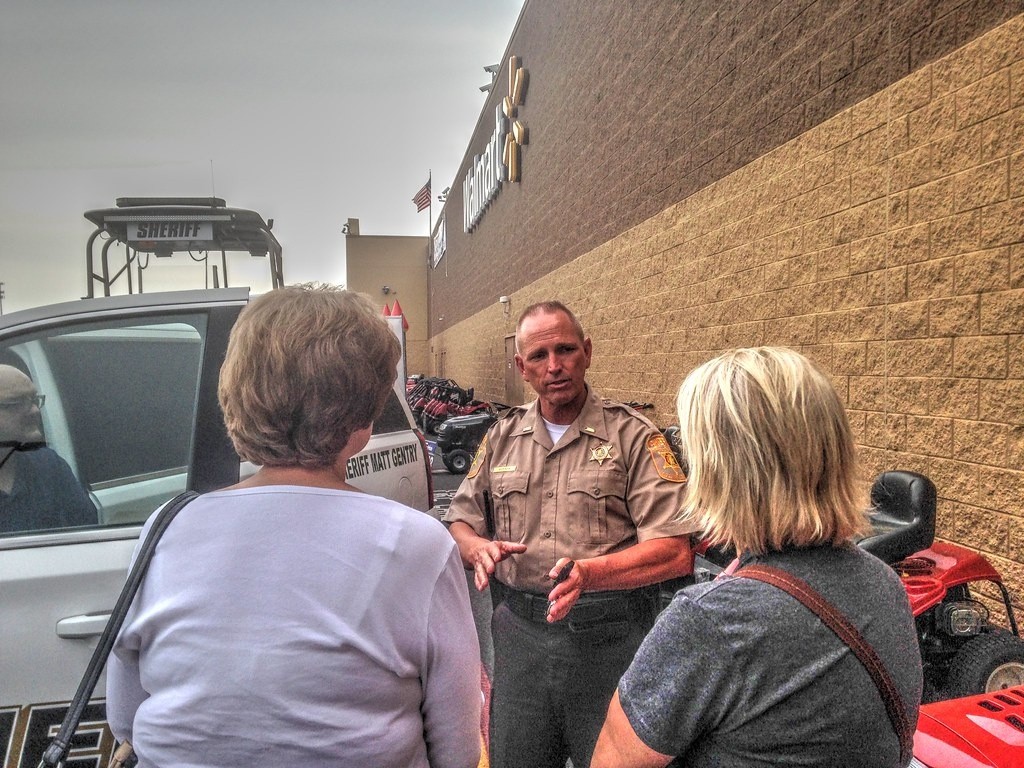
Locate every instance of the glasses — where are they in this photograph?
[0,395,45,409]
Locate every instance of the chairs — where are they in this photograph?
[854,470,937,565]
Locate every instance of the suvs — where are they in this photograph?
[0,286,438,768]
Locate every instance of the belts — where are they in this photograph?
[502,588,658,624]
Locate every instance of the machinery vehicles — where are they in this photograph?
[655,469,1023,700]
[907,684,1023,768]
[406,373,510,473]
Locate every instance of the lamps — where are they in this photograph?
[437,186,450,202]
[499,295,510,316]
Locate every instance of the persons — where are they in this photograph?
[106,286,482,768]
[441,302,697,768]
[589,346,923,768]
[0,364,98,533]
